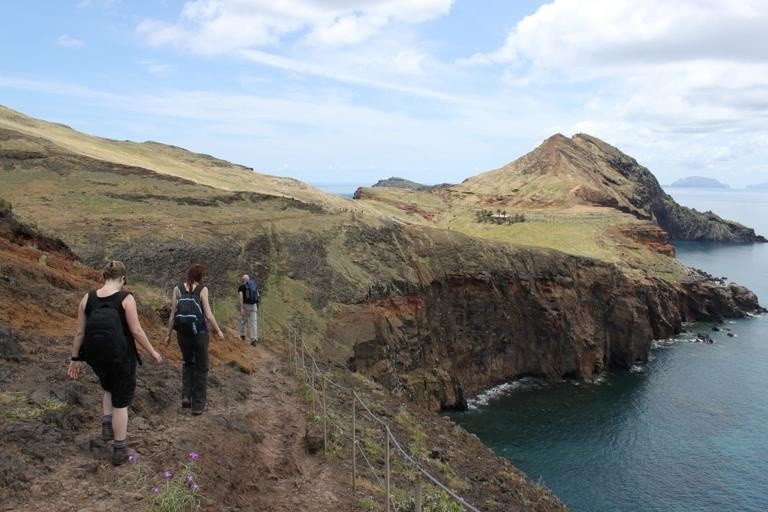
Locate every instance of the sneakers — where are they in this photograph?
[240,336,257,346]
[182,398,204,414]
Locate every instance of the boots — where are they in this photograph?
[113,446,129,464]
[102,420,114,440]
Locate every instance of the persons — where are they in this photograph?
[237,273,261,347]
[67,261,162,466]
[165,263,223,414]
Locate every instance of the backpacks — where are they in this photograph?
[174,282,207,337]
[244,281,260,302]
[85,287,132,366]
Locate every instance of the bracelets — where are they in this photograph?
[72,356,80,361]
